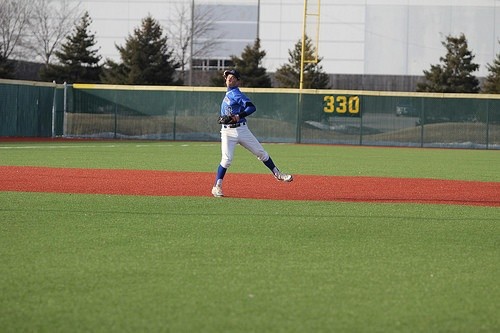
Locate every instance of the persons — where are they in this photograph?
[211,70,293,198]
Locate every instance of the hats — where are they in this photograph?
[224,69,240,81]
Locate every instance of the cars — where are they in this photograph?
[395,97,411,117]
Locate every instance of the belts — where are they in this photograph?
[224,123,245,128]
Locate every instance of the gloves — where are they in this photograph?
[217,115,235,124]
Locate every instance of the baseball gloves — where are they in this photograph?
[217,114,237,124]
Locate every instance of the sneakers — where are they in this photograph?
[274,172,293,182]
[211,186,223,198]
